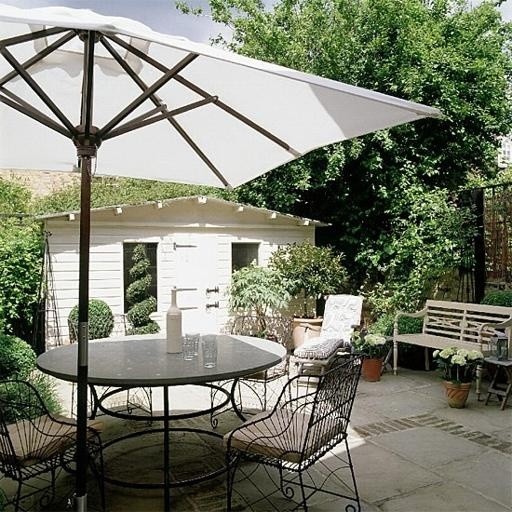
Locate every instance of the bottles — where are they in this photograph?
[166,289,182,354]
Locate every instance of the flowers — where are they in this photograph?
[432,345,483,385]
[351,331,386,358]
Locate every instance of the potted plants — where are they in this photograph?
[267,240,350,346]
[231,264,290,343]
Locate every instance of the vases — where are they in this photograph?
[443,380,471,408]
[363,359,382,382]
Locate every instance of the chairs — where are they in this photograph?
[1,368,105,512]
[393,299,512,402]
[67,314,153,426]
[224,354,363,512]
[289,294,363,387]
[210,335,293,429]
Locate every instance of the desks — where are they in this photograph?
[36,334,286,511]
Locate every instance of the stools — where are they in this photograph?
[484,355,512,410]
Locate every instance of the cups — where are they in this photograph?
[202,342,217,369]
[186,333,200,356]
[181,337,195,361]
[202,334,215,341]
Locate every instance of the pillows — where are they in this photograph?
[294,337,342,358]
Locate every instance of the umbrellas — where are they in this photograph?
[0,2,440,511]
